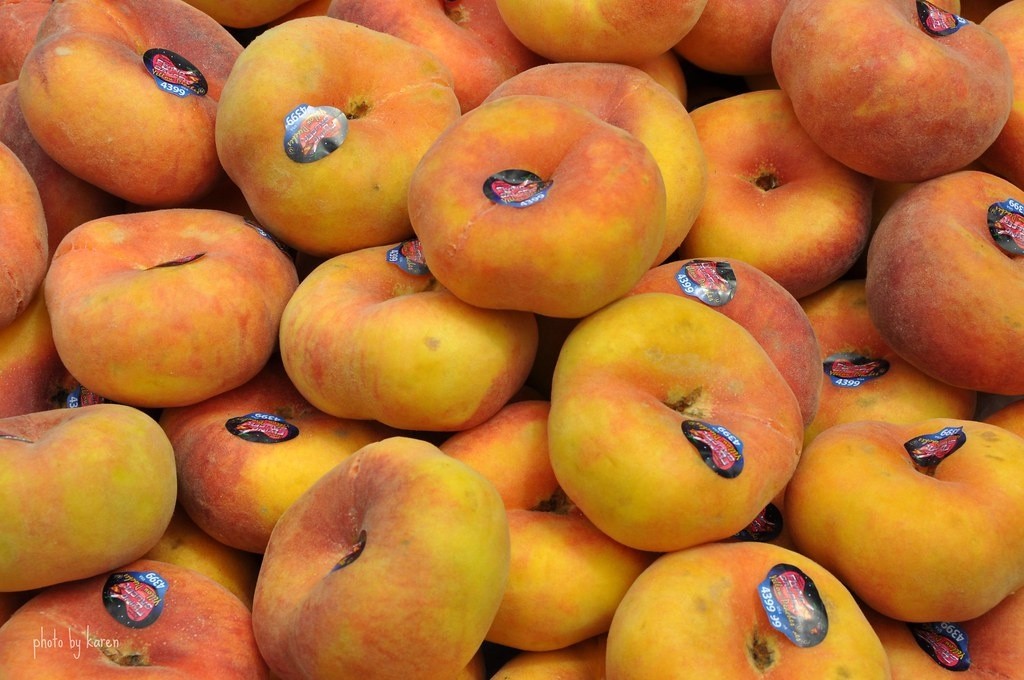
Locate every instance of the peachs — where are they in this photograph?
[0,0,1024,680]
